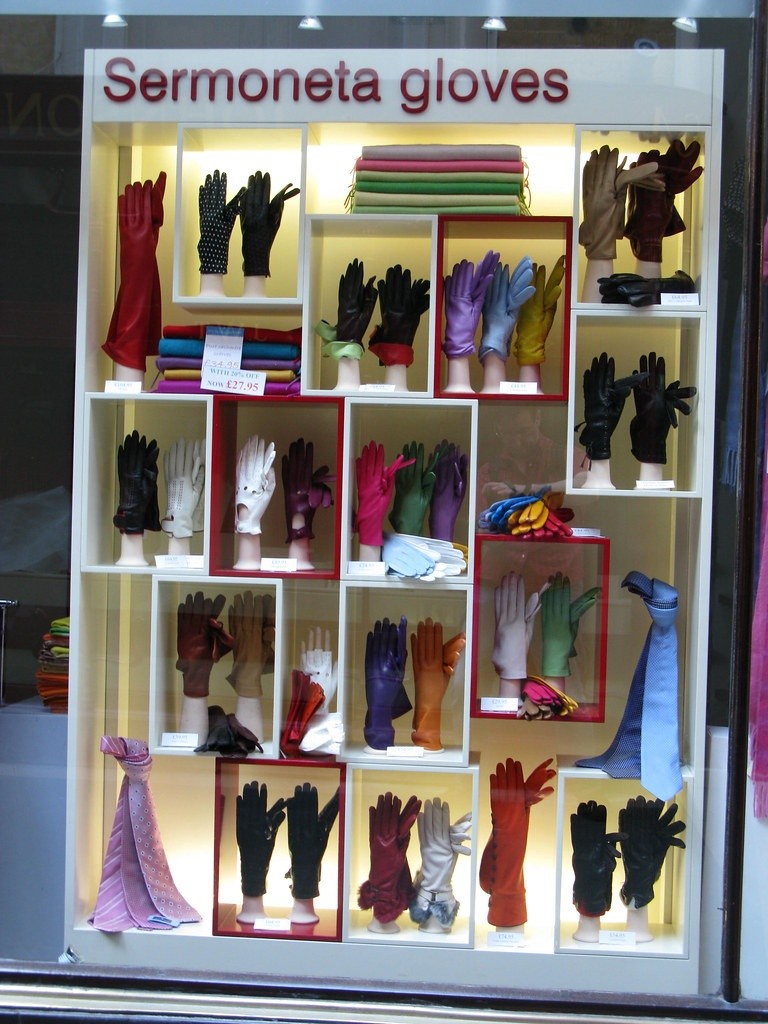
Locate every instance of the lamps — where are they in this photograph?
[671,18,697,33]
[100,14,128,28]
[298,15,325,31]
[482,17,507,32]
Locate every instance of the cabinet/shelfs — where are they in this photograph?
[78,116,709,962]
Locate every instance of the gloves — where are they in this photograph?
[236,780,339,900]
[443,250,566,367]
[479,757,557,927]
[197,168,300,279]
[353,439,469,583]
[357,792,472,928]
[541,572,602,679]
[233,433,335,544]
[316,257,430,368]
[597,270,694,307]
[579,139,704,263]
[101,170,168,372]
[490,571,550,680]
[175,590,275,759]
[113,430,206,539]
[477,486,576,539]
[569,800,630,918]
[574,351,697,472]
[517,675,578,722]
[617,794,686,910]
[362,615,466,754]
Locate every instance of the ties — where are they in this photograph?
[87,735,203,934]
[576,572,684,801]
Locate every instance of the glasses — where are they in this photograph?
[493,416,536,442]
